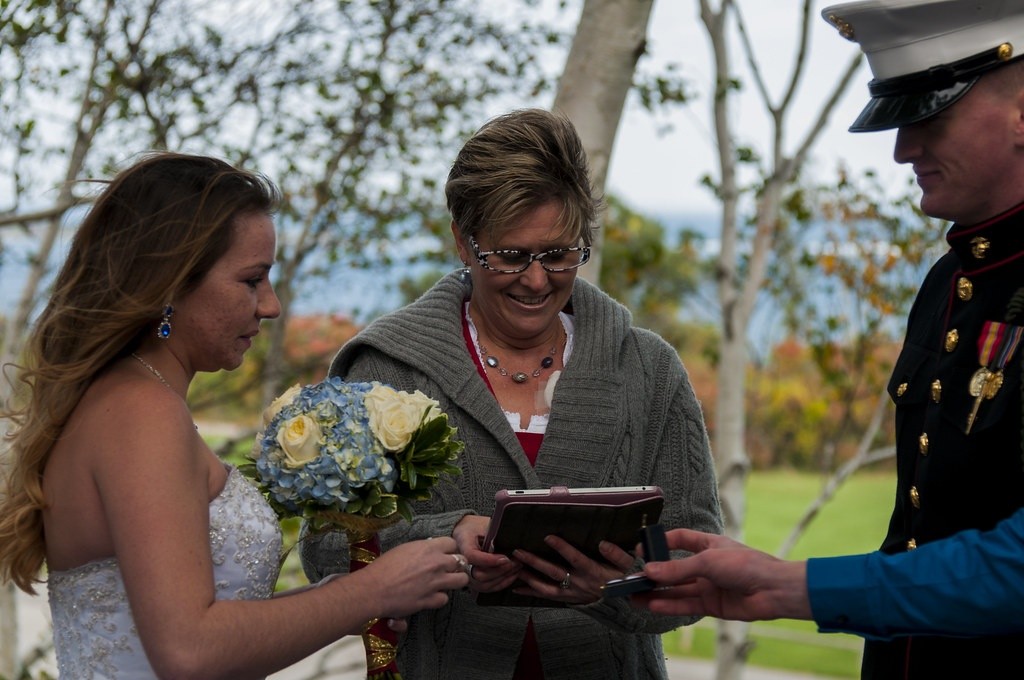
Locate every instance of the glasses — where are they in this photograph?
[466,231,592,274]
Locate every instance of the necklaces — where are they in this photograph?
[130,352,199,430]
[475,337,565,384]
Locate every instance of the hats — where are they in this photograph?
[821,0,1024,133]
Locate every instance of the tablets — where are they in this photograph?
[475,486,664,609]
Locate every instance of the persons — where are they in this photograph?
[627,0,1024,680]
[0,155,468,680]
[298,110,724,680]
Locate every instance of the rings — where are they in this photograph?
[452,555,466,573]
[465,564,473,578]
[560,572,570,589]
